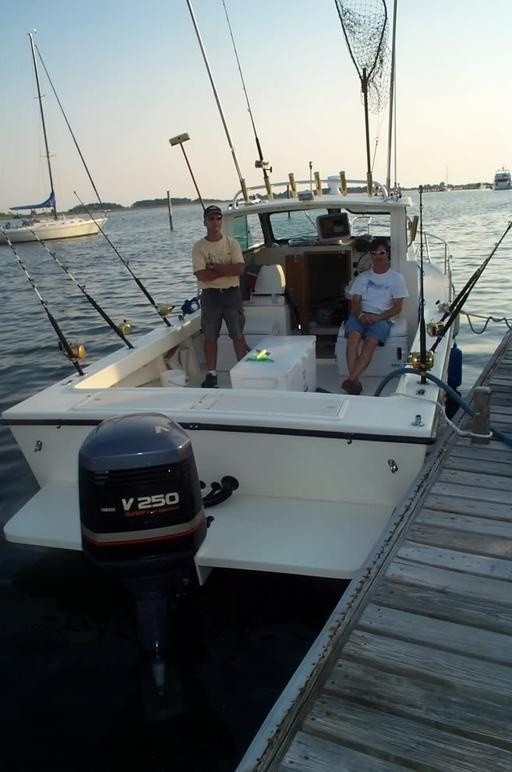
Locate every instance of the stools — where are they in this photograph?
[213,319,277,370]
[336,318,409,378]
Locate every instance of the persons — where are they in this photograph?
[192,205,247,388]
[340,238,410,395]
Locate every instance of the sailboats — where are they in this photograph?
[1,28,108,247]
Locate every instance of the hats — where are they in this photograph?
[204,205,223,218]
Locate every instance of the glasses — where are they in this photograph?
[209,217,222,221]
[369,250,386,257]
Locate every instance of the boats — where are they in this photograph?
[478,183,491,191]
[494,172,512,189]
[2,0,465,582]
[444,186,454,192]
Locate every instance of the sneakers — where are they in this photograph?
[202,373,217,388]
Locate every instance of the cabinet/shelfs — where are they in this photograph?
[229,336,317,391]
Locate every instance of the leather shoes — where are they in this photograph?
[341,379,359,394]
[354,382,363,395]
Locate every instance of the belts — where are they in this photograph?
[218,288,228,293]
